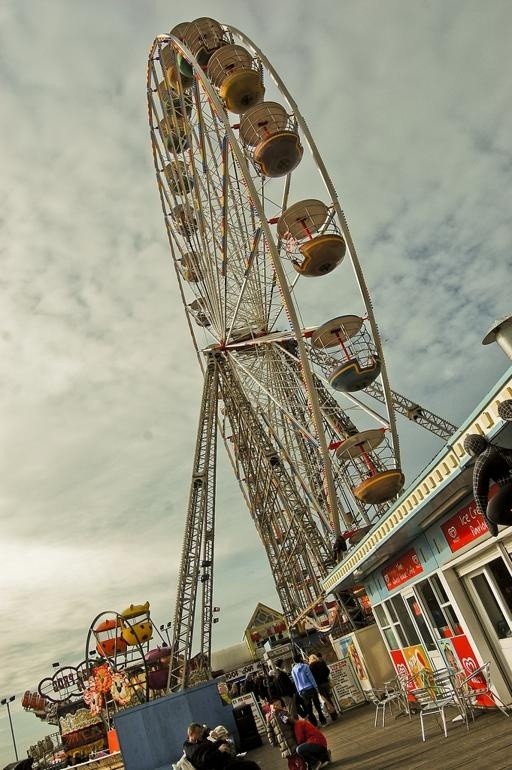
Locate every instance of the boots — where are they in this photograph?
[328,711,340,721]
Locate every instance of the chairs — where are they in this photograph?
[362,659,511,742]
[171,750,197,770]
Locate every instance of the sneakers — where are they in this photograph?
[313,721,330,729]
[312,760,322,770]
[321,760,329,769]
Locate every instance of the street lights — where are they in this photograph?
[52,662,68,696]
[0,696,19,761]
[89,650,96,657]
[159,622,172,647]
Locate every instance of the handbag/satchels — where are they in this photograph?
[316,693,335,723]
[328,677,337,690]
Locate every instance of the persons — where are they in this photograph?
[441,643,473,710]
[180,652,340,769]
